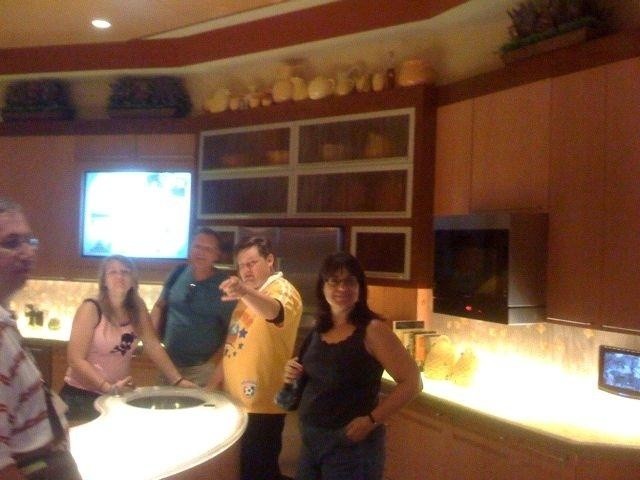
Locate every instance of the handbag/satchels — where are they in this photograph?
[14,380,84,480]
[272,328,315,412]
[157,263,188,341]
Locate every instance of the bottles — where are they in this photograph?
[203,52,435,113]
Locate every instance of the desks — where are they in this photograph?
[377,378,640,480]
[22,338,225,393]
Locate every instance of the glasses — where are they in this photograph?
[2,235,40,250]
[328,278,357,286]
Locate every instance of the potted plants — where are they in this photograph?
[499,0,615,59]
[0,78,76,120]
[106,75,192,117]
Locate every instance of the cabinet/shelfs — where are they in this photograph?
[191,84,438,288]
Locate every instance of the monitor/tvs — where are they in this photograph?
[597,345,640,400]
[83,171,191,259]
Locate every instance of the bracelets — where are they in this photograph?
[172,377,184,387]
[111,383,120,396]
[366,413,383,429]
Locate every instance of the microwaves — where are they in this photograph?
[432,229,508,327]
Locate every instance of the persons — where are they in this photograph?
[56,253,201,429]
[0,195,71,480]
[139,225,239,385]
[283,250,425,480]
[202,234,303,480]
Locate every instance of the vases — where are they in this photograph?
[206,58,438,114]
